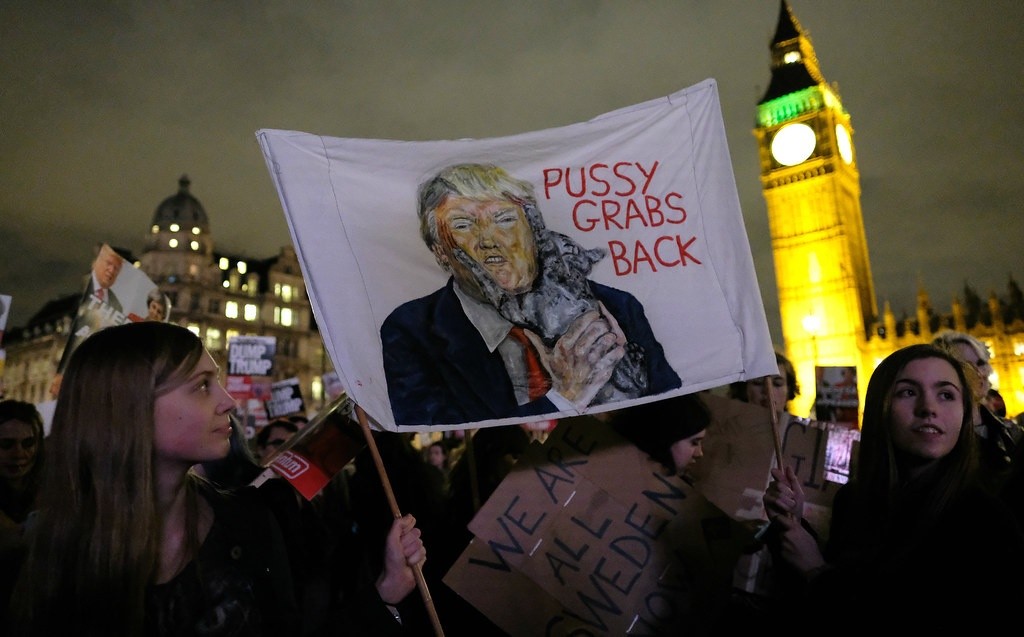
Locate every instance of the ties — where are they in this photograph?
[97,288,104,300]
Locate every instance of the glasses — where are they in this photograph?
[260,438,286,449]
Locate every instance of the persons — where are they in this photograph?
[125,288,168,323]
[0,391,714,637]
[722,343,1024,637]
[55,240,128,376]
[0,319,317,637]
